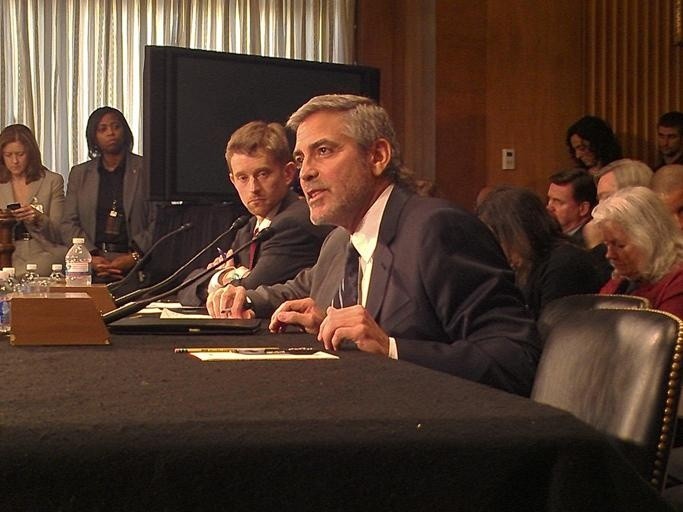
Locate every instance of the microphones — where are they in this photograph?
[99,225,276,323]
[111,213,251,304]
[107,196,238,295]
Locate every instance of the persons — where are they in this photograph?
[205,165,437,320]
[176,121,334,308]
[268,94,543,398]
[1,123,65,277]
[477,109,683,318]
[57,106,163,288]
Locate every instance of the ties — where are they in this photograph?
[328,244,360,308]
[249,228,259,270]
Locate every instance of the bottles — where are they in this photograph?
[64,235,92,289]
[0,264,66,333]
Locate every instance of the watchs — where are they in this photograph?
[132,251,141,263]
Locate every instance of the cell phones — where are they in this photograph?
[6,202,21,211]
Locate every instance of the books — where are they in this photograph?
[106,313,262,335]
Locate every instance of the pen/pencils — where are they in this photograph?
[221,304,253,312]
[174,347,321,354]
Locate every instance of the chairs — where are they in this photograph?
[520,303,682,510]
[528,290,653,364]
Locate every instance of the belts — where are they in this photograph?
[97,242,127,252]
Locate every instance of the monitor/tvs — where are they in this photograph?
[140,42,386,201]
